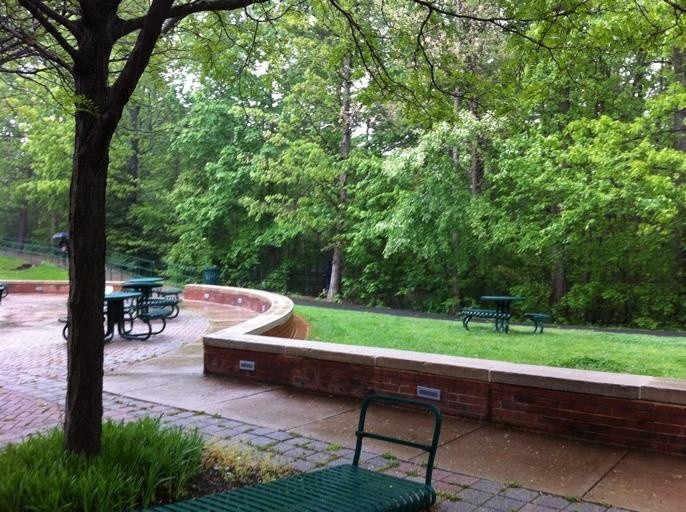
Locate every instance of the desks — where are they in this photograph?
[480,294,528,332]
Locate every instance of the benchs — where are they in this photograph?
[517,312,551,332]
[128,393,446,512]
[60,274,181,341]
[456,308,511,331]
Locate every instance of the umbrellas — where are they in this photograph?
[50,231,71,248]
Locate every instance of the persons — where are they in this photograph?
[59,238,67,253]
